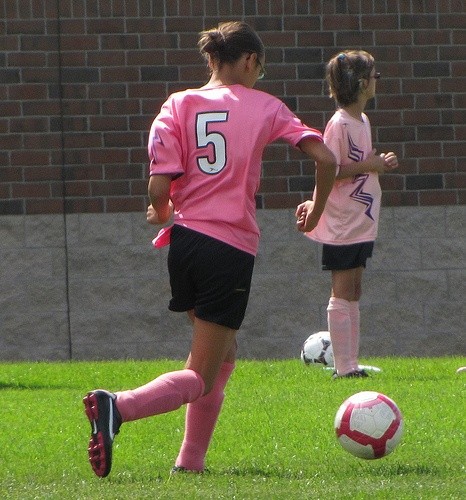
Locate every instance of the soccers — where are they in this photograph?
[334,391,403,460]
[301,331,334,368]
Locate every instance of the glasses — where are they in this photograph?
[246,49,267,79]
[363,72,382,79]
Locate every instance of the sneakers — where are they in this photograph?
[332,370,371,380]
[169,465,212,482]
[83,389,122,478]
[331,369,368,376]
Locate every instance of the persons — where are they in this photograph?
[303,49,400,382]
[82,20,338,484]
